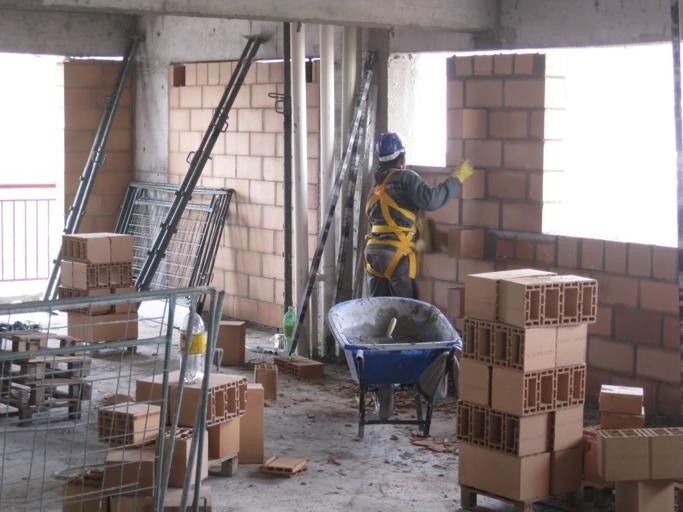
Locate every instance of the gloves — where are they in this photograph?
[452,158,474,183]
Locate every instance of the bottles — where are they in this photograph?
[178,305,204,385]
[282,305,298,354]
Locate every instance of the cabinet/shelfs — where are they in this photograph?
[0,329,93,427]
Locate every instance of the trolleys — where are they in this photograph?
[326,296,462,438]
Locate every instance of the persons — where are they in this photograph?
[362,129,474,390]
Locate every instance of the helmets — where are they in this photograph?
[376,133,405,163]
